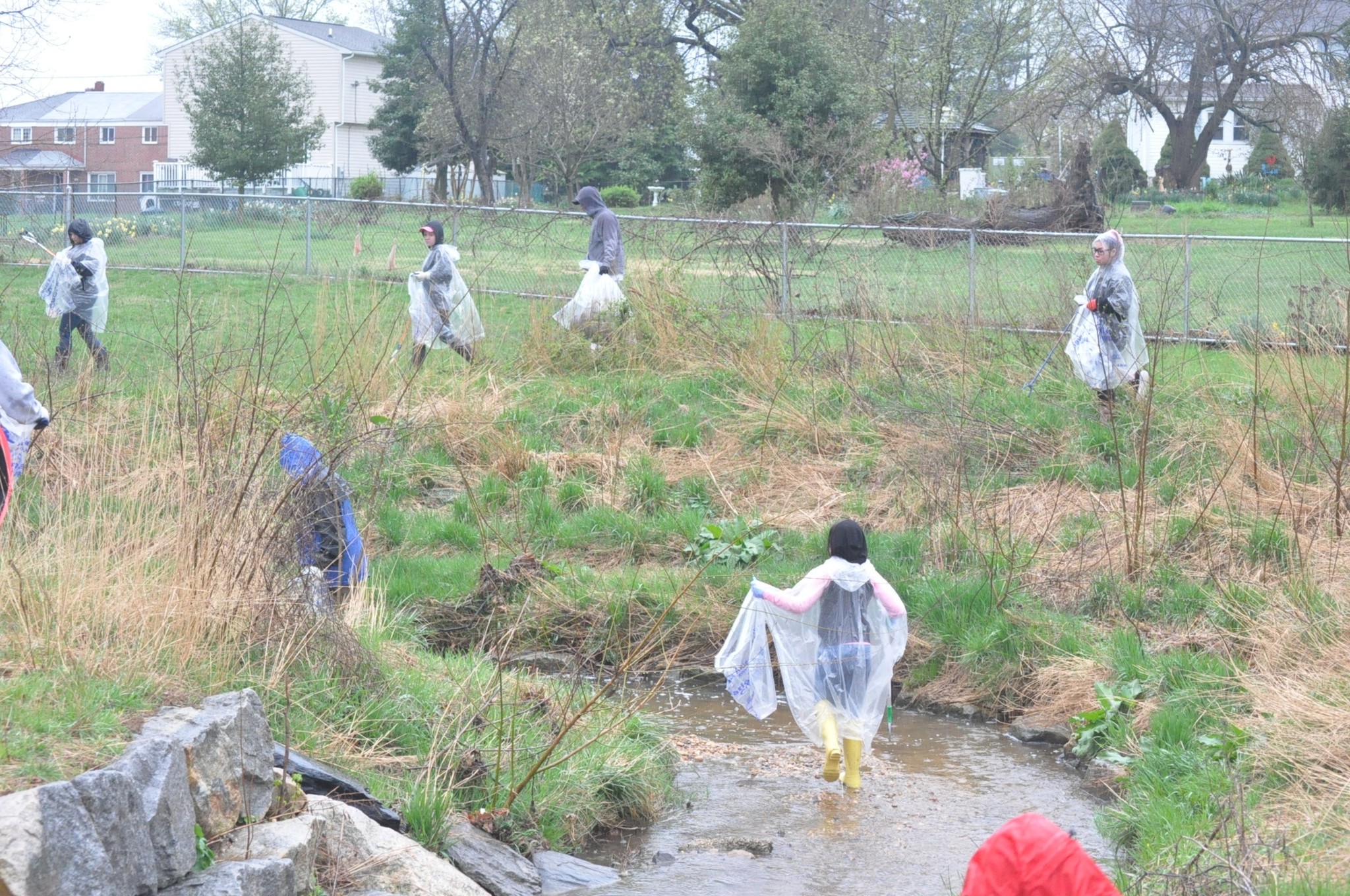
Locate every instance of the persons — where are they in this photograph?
[572,186,625,342]
[1084,228,1150,400]
[0,337,50,521]
[411,220,492,370]
[750,518,907,790]
[49,218,110,373]
[279,432,371,612]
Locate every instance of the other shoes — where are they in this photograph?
[1133,367,1152,403]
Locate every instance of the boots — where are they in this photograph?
[52,346,74,374]
[839,718,864,788]
[813,701,846,783]
[410,347,427,372]
[453,340,489,369]
[89,345,111,372]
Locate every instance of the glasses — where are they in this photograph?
[1090,247,1115,255]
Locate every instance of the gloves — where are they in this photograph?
[1086,298,1096,311]
[411,270,431,283]
[34,407,50,430]
[748,579,766,599]
[599,265,611,274]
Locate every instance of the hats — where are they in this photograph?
[571,198,579,205]
[419,226,435,233]
